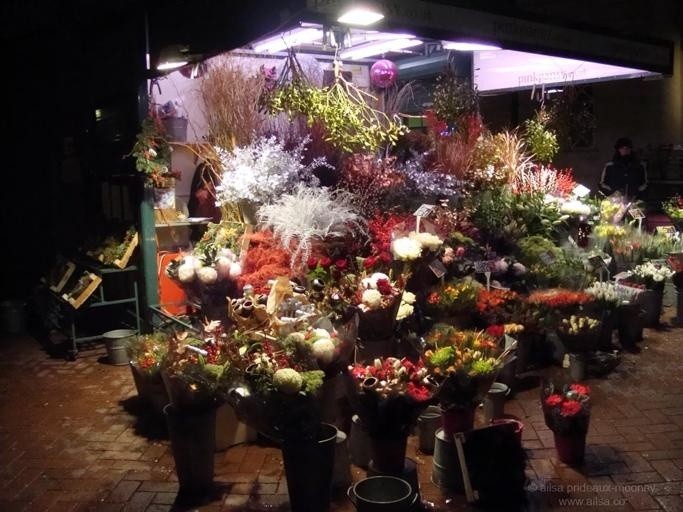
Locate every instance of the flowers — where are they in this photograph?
[132,134,681,428]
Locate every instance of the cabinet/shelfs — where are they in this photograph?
[38,251,138,349]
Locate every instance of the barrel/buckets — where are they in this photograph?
[334,431,356,497]
[482,381,511,421]
[345,473,420,510]
[417,404,442,456]
[431,430,463,494]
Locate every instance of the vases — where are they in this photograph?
[158,407,593,511]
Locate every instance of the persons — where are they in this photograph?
[599,137,650,202]
